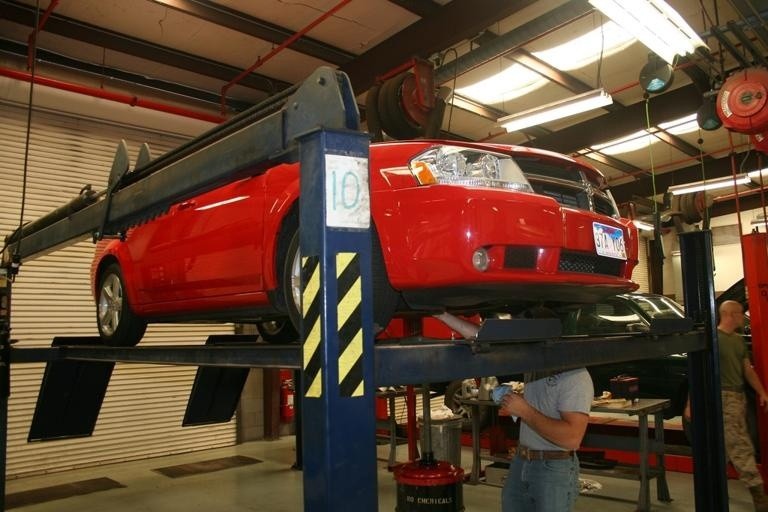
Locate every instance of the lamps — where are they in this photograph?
[497,87,613,133]
[667,172,751,196]
[587,0,711,65]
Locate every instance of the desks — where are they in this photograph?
[376,388,423,467]
[461,399,670,512]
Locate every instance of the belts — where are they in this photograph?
[722,385,747,394]
[518,448,574,460]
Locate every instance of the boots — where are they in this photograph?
[749,483,768,512]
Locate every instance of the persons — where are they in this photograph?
[432,304,594,512]
[683,299,767,511]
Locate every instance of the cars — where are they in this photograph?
[498,277,758,420]
[90,138,639,347]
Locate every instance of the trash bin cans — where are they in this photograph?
[417,409,462,468]
